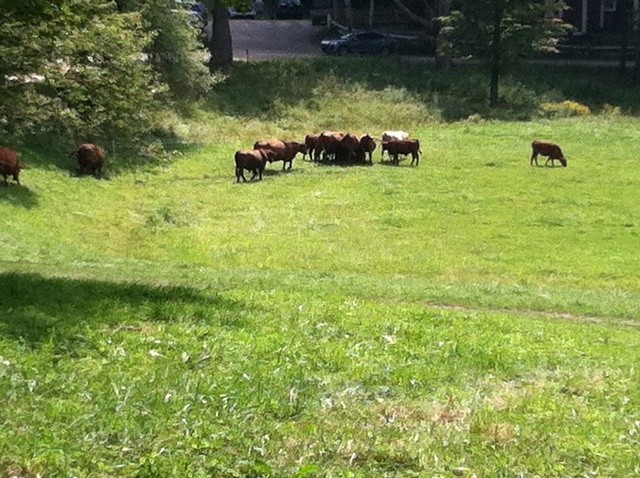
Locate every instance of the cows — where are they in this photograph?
[235,149,279,183]
[530,140,567,167]
[319,132,344,161]
[382,131,410,159]
[360,133,376,163]
[341,134,359,162]
[303,131,320,159]
[381,140,421,166]
[69,144,107,179]
[0,147,25,185]
[254,140,306,170]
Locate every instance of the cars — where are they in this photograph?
[271,0,304,19]
[321,30,396,57]
